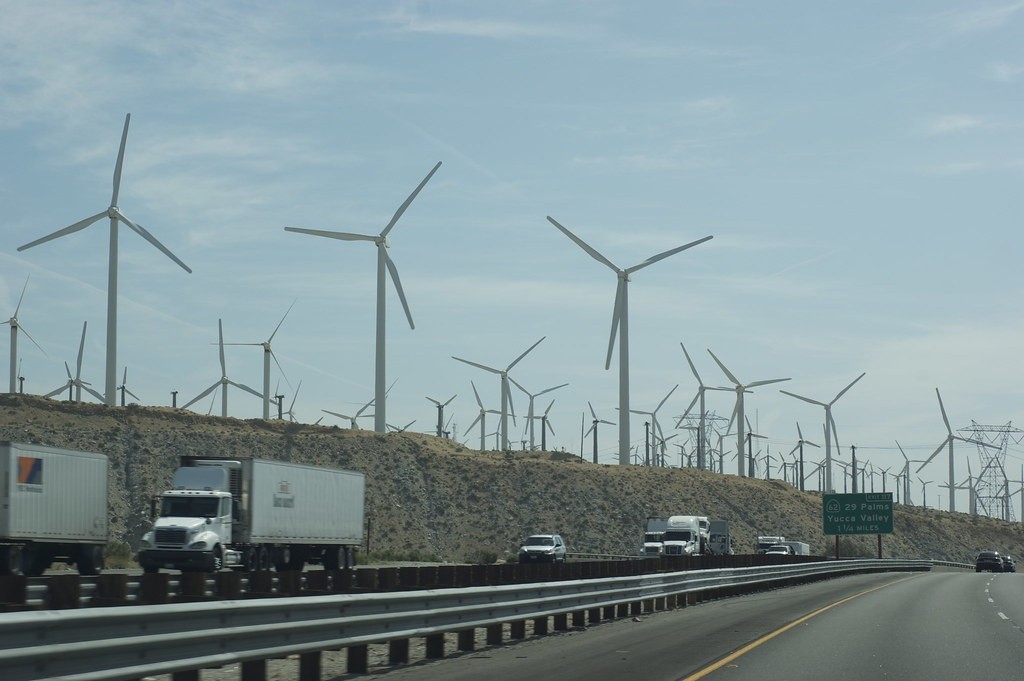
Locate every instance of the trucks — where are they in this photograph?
[134,454,366,571]
[756,536,785,555]
[0,440,111,574]
[779,541,810,555]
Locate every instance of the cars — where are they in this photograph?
[765,545,795,555]
[974,551,1003,573]
[1001,556,1016,573]
[518,534,566,564]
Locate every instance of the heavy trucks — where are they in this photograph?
[640,519,728,556]
[663,516,713,556]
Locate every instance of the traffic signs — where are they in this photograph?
[823,492,892,534]
